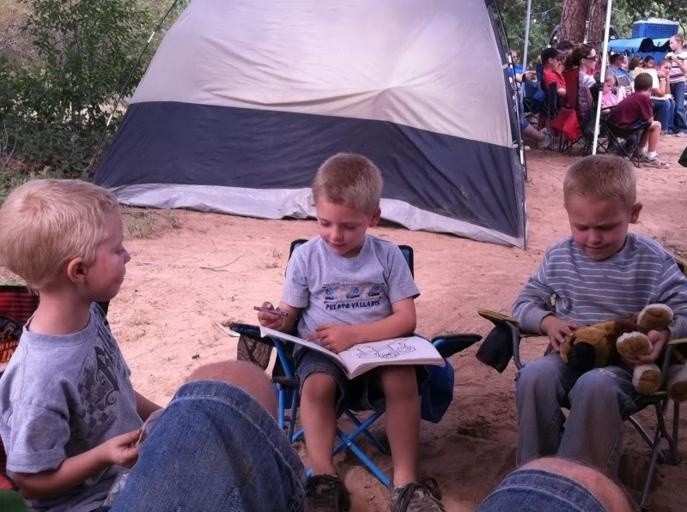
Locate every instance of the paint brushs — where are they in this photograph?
[253,305,289,317]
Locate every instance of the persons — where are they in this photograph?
[508,30,686,167]
[1,175,167,511]
[253,149,452,512]
[505,153,685,481]
[106,356,637,512]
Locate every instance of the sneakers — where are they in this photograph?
[643,155,669,169]
[307,473,351,512]
[538,130,554,149]
[630,149,647,162]
[388,476,444,511]
[659,131,686,138]
[539,127,548,136]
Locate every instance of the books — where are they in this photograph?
[216,309,447,378]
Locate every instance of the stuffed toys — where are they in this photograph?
[560,303,684,403]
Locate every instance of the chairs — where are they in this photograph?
[532,64,650,169]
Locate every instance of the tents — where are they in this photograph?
[75,1,526,248]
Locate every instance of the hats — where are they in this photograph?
[540,48,569,63]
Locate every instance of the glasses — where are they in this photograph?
[584,54,599,62]
